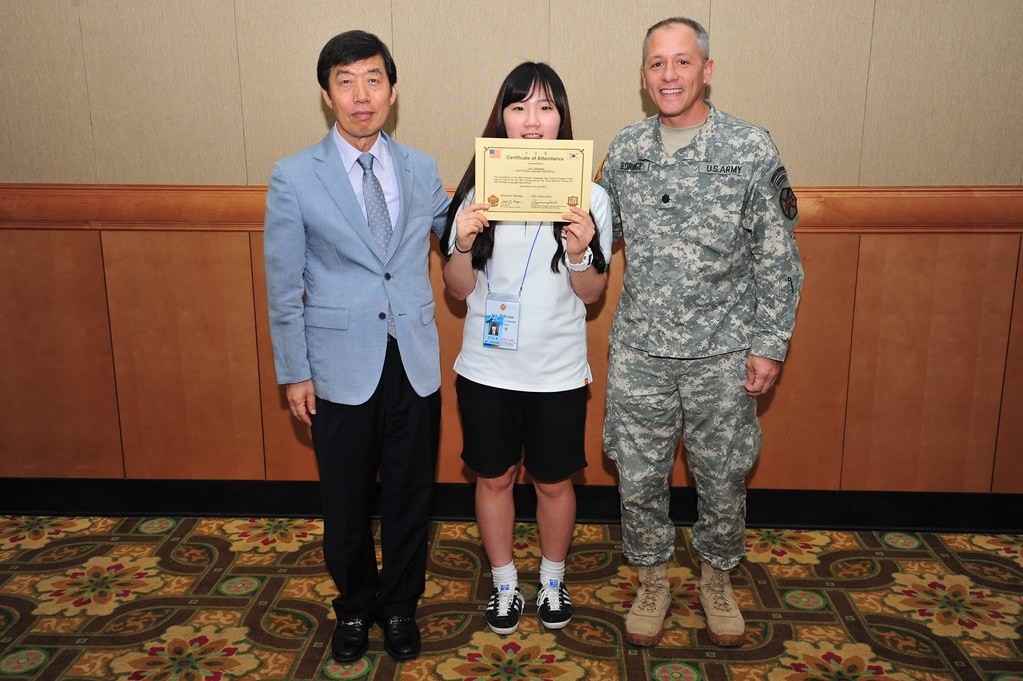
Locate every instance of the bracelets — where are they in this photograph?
[455,237,473,254]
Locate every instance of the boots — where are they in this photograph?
[698,560,746,646]
[624,562,672,646]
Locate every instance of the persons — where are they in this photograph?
[439,62,612,633]
[593,16,804,646]
[263,29,452,664]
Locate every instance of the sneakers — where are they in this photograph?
[535,578,573,628]
[484,585,525,634]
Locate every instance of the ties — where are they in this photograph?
[355,153,398,341]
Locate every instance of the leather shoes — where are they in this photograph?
[331,615,369,663]
[375,609,421,662]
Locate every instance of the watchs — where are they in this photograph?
[565,246,593,272]
